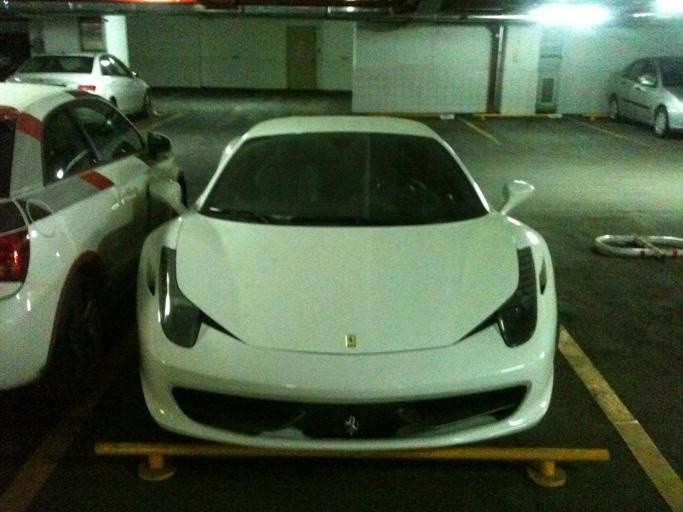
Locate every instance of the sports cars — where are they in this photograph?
[136,112,562,455]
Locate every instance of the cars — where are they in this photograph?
[605,54,682,138]
[7,52,159,120]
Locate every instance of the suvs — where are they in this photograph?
[0,78,189,402]
